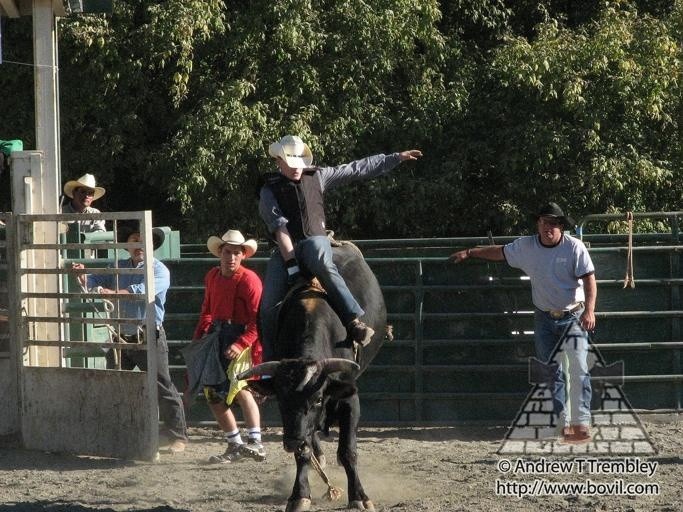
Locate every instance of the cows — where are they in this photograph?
[235,240,389,511]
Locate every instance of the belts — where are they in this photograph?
[113,333,139,343]
[536,304,582,320]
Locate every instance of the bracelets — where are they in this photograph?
[466,248,471,257]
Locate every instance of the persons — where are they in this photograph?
[61,173,107,258]
[179,229,267,461]
[258,135,424,363]
[448,202,598,441]
[69,225,187,455]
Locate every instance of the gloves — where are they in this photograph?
[285,257,302,282]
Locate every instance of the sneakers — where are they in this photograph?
[346,322,375,349]
[209,442,242,463]
[238,440,266,462]
[168,441,187,453]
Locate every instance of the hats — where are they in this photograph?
[527,201,576,231]
[207,230,258,259]
[269,135,313,168]
[120,220,165,251]
[63,174,105,200]
